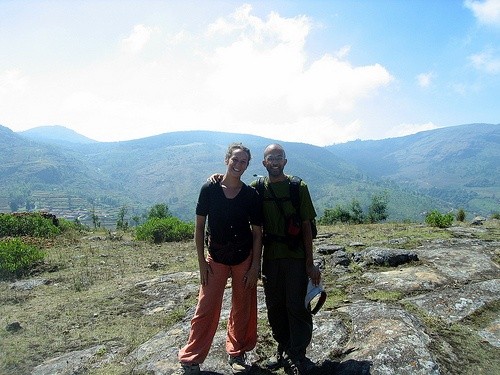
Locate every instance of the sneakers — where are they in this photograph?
[227,355,246,374]
[181,363,200,375]
[266,354,281,369]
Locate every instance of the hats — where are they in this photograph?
[305,279,327,315]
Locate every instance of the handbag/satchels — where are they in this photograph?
[285,215,304,250]
[207,242,250,266]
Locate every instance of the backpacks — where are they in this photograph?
[256,175,317,239]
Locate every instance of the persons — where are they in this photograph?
[178,142,263,375]
[207,144,321,375]
